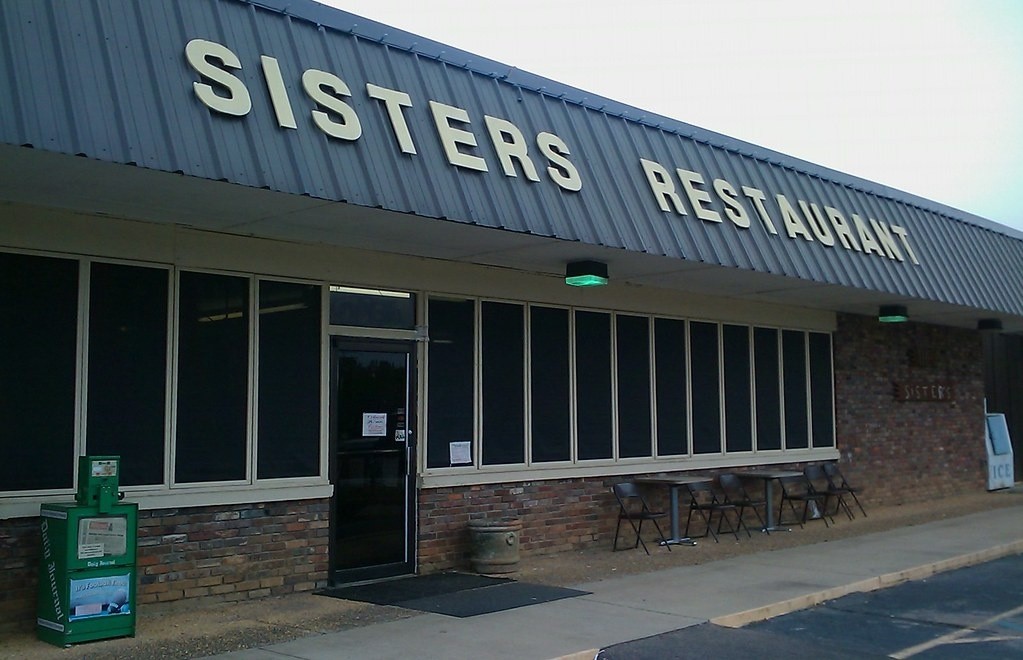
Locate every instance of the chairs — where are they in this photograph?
[613,463,867,556]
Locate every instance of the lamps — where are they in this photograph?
[978,319,1004,331]
[878,305,908,322]
[565,260,608,289]
[196,299,311,324]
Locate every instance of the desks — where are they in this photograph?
[733,470,803,532]
[633,476,714,546]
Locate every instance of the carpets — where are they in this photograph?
[396,581,594,619]
[312,572,517,606]
